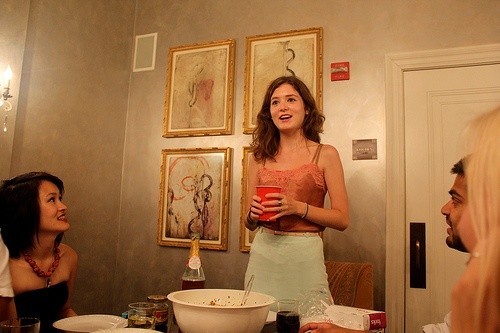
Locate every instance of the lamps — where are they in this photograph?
[0,63,14,133]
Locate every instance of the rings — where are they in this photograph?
[278,201,282,205]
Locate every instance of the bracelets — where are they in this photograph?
[303,202,309,221]
[246,211,254,224]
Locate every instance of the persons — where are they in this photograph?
[299,105,500,333]
[241,76,350,312]
[0,172,77,333]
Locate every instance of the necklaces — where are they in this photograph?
[23,259,64,285]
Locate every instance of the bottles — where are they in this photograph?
[182,233,206,291]
[147,294,167,333]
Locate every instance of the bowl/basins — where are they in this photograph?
[167,289,276,333]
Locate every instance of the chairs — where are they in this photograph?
[320,261,374,308]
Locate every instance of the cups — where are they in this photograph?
[0,317,40,333]
[255,186,282,221]
[129,302,154,329]
[276,299,300,333]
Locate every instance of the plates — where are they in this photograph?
[95,328,163,333]
[52,314,128,333]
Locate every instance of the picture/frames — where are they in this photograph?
[161,36,238,139]
[242,25,326,137]
[154,146,236,253]
[239,144,261,254]
